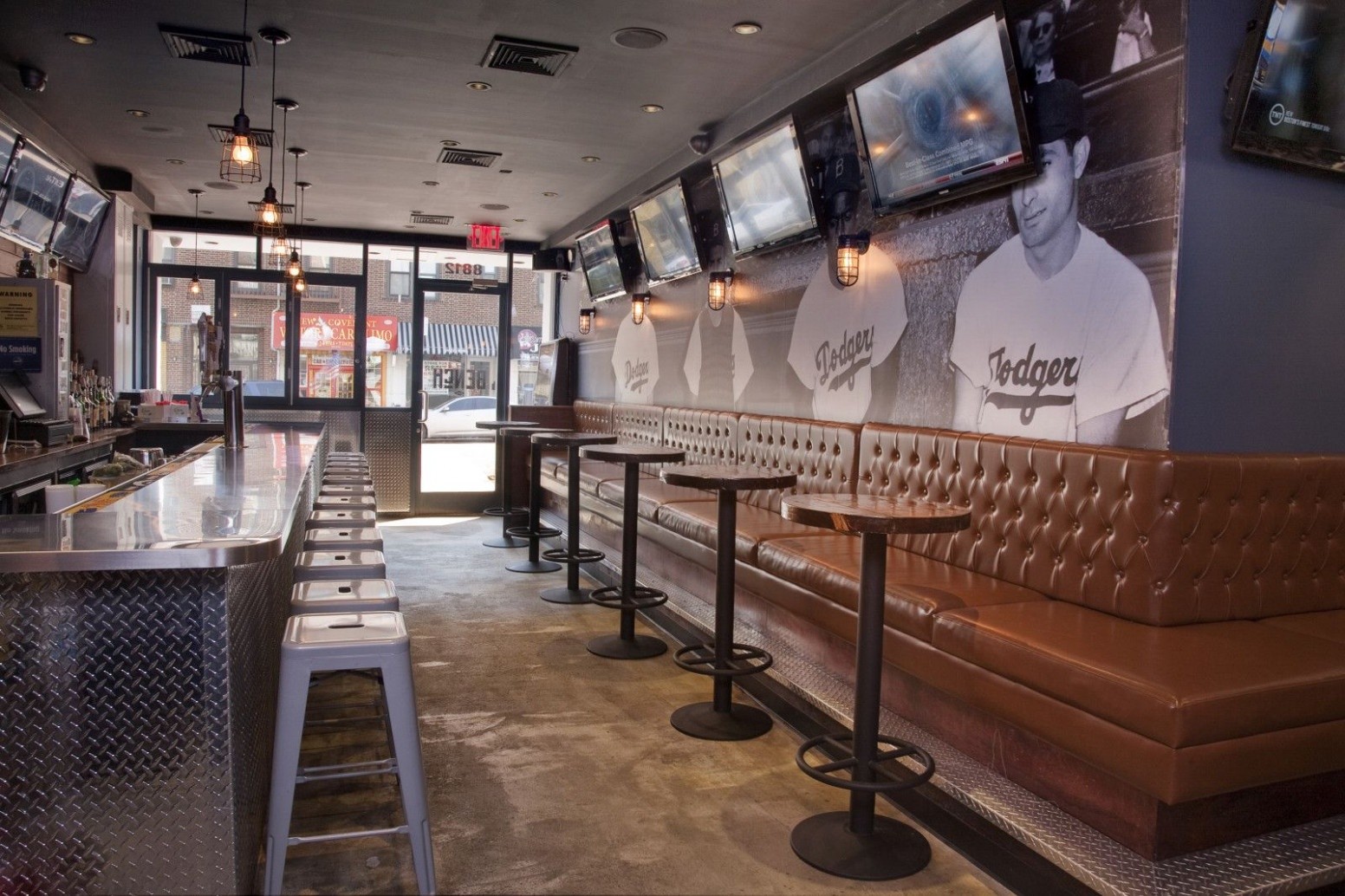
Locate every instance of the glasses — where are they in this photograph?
[1032,23,1054,38]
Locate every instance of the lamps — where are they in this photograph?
[253,27,292,238]
[219,1,262,183]
[632,290,650,325]
[579,307,597,335]
[269,97,312,300]
[186,189,206,300]
[709,268,734,311]
[835,231,871,286]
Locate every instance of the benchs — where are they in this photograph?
[505,400,1343,863]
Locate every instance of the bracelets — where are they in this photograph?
[1137,28,1149,41]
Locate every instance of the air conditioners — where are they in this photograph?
[161,277,173,285]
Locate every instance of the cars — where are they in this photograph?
[422,395,500,440]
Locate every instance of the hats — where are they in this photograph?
[819,152,861,202]
[1023,79,1085,146]
[702,222,727,257]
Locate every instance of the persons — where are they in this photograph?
[1111,1,1156,74]
[683,238,755,413]
[1021,3,1095,98]
[785,152,910,425]
[948,81,1171,452]
[612,262,659,406]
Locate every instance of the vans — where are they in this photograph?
[185,380,305,396]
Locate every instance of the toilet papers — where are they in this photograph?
[75,484,106,502]
[44,484,77,514]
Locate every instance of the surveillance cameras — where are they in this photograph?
[19,66,48,93]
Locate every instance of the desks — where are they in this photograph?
[531,432,617,605]
[476,421,540,548]
[579,444,685,660]
[499,427,574,574]
[659,464,798,744]
[781,492,971,880]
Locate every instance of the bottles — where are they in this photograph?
[68,361,114,442]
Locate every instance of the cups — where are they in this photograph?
[44,483,106,514]
[129,447,176,472]
[0,409,14,454]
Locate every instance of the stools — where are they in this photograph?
[264,611,439,896]
[289,452,400,773]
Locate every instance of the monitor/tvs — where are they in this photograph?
[844,0,1042,222]
[0,109,114,274]
[575,218,630,302]
[709,112,827,262]
[1224,0,1345,173]
[629,177,707,288]
